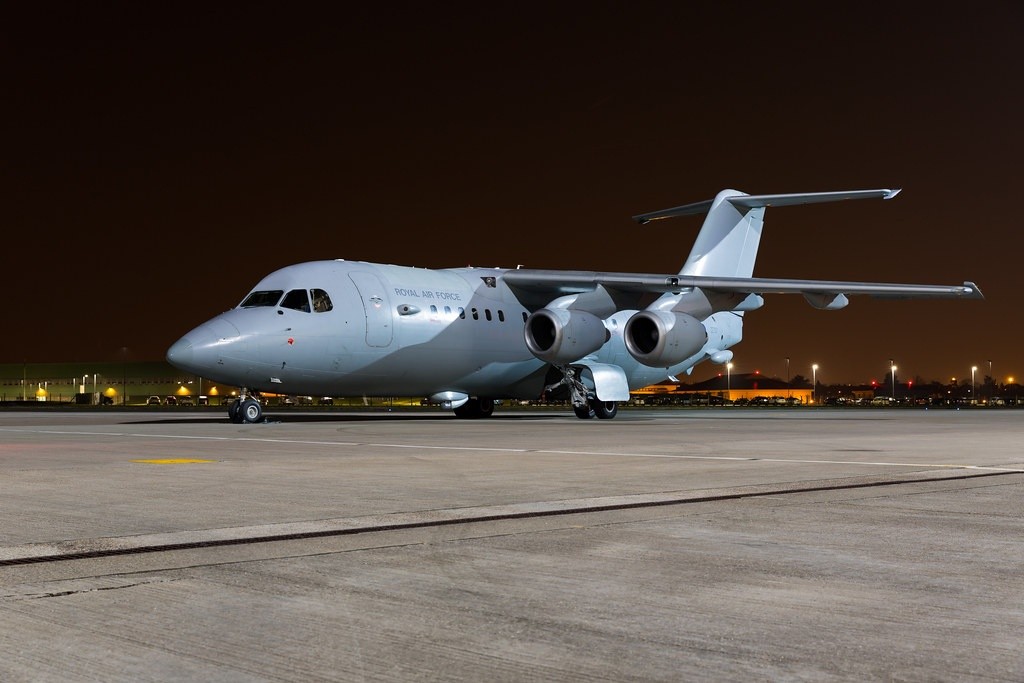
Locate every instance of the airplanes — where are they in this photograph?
[166,184,986,425]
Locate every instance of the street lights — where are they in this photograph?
[812,363,819,398]
[786,357,791,398]
[726,362,733,391]
[892,365,898,398]
[971,366,978,400]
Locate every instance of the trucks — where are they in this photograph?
[75,392,114,405]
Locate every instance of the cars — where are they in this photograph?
[420,395,1024,412]
[318,396,333,406]
[144,396,161,406]
[179,395,193,407]
[163,396,177,406]
[278,395,295,407]
[297,396,314,405]
